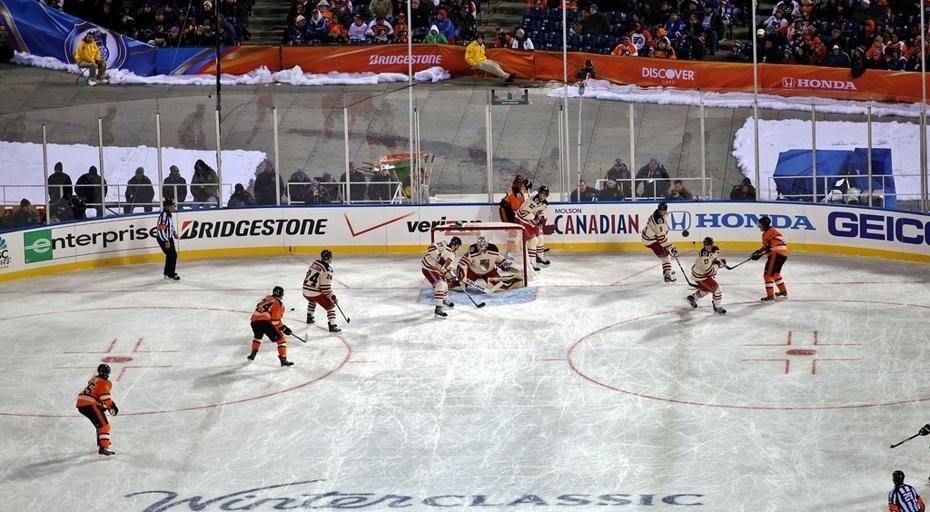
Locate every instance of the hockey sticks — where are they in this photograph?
[674,255,698,287]
[334,299,351,323]
[726,253,759,270]
[456,280,486,308]
[889,431,921,449]
[291,333,309,344]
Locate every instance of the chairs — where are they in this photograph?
[341,18,352,28]
[575,34,600,55]
[463,53,487,80]
[546,7,563,22]
[470,1,483,25]
[543,32,562,51]
[561,9,578,25]
[387,17,394,24]
[636,44,649,56]
[843,22,859,35]
[605,12,621,26]
[416,25,431,36]
[525,7,544,21]
[74,50,93,83]
[0,205,15,228]
[561,33,578,52]
[673,38,693,61]
[888,13,911,25]
[352,3,369,14]
[611,21,627,36]
[518,17,539,35]
[897,26,911,39]
[362,15,374,22]
[36,204,46,224]
[600,34,617,54]
[527,31,544,50]
[537,19,555,32]
[560,21,574,32]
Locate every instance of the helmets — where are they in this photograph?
[658,202,668,210]
[759,217,771,227]
[321,249,332,260]
[98,364,110,377]
[451,237,461,245]
[538,186,549,197]
[703,237,713,245]
[477,236,488,250]
[513,175,525,186]
[273,286,284,297]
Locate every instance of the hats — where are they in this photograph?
[164,200,175,207]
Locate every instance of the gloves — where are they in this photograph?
[280,325,292,335]
[667,245,678,256]
[752,250,761,260]
[718,259,727,267]
[331,294,336,302]
[109,403,118,416]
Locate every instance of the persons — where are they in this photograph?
[665,179,693,203]
[512,29,534,50]
[455,237,523,296]
[247,286,294,367]
[499,174,532,222]
[465,33,517,84]
[74,167,108,217]
[91,0,255,48]
[535,29,610,54]
[309,172,340,203]
[422,235,462,317]
[157,201,183,282]
[653,0,745,61]
[75,32,108,86]
[0,207,18,228]
[76,364,118,456]
[750,216,789,302]
[610,37,638,57]
[253,161,285,205]
[162,166,187,208]
[583,4,609,33]
[348,0,482,45]
[609,0,653,26]
[609,25,629,37]
[123,167,154,213]
[604,157,631,199]
[514,185,550,271]
[229,183,254,206]
[628,21,653,57]
[190,158,220,205]
[640,201,679,281]
[368,164,398,203]
[740,0,930,72]
[684,237,730,316]
[282,0,352,45]
[303,249,342,332]
[626,159,671,201]
[518,1,587,30]
[494,27,514,48]
[283,168,313,204]
[339,161,366,202]
[729,177,756,201]
[596,179,624,203]
[888,471,926,512]
[569,180,598,203]
[47,162,72,199]
[14,200,41,226]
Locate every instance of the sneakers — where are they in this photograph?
[168,274,180,280]
[164,272,177,276]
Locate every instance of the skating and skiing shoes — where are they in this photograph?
[328,322,341,335]
[713,305,727,314]
[307,314,315,325]
[247,351,257,362]
[684,295,697,308]
[278,355,294,369]
[761,296,774,304]
[530,261,540,272]
[774,291,787,299]
[97,437,111,446]
[99,447,115,458]
[664,275,678,282]
[663,270,675,275]
[536,254,550,266]
[443,299,454,309]
[434,306,448,318]
